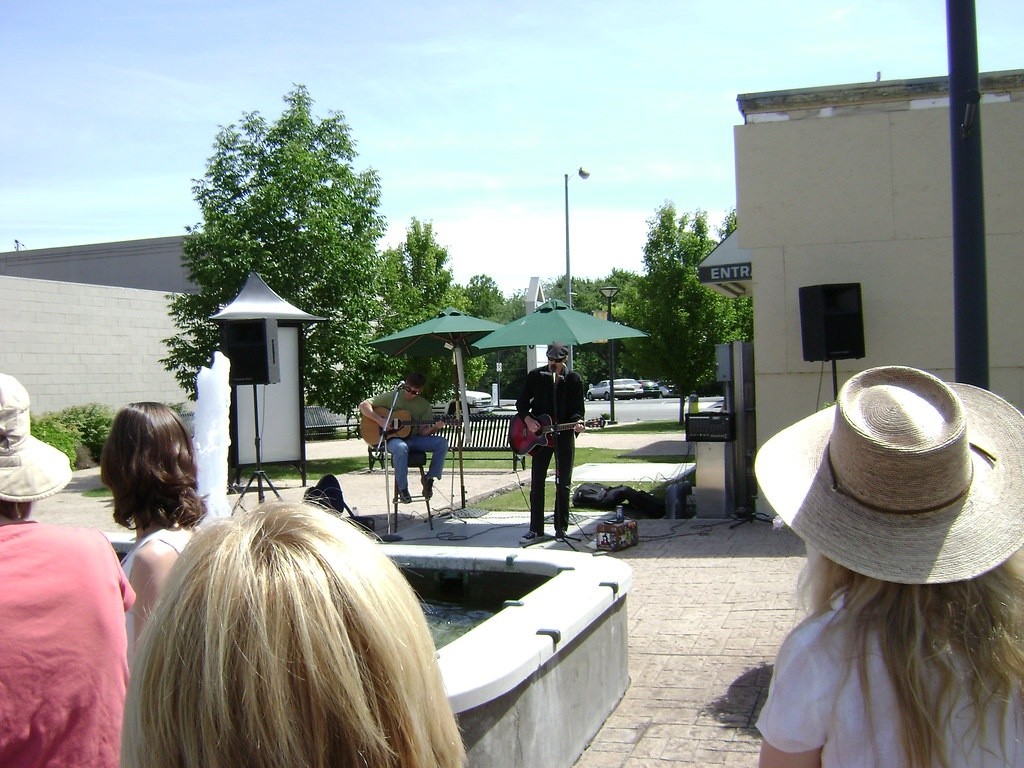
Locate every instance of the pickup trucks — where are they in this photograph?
[431,390,491,414]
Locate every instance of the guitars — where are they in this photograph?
[509,413,606,457]
[361,407,460,445]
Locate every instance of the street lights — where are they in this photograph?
[564,167,591,372]
[600,286,622,424]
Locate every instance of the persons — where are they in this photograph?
[357,374,448,504]
[0,371,137,768]
[119,495,474,767]
[515,342,586,544]
[101,401,209,768]
[753,366,1024,768]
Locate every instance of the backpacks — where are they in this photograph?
[572,482,641,512]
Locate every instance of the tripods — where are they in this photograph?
[230,384,285,517]
[729,455,773,528]
[522,383,582,552]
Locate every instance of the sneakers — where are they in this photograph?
[520,531,545,541]
[555,530,565,541]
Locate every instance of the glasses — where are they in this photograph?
[546,356,565,363]
[405,383,421,395]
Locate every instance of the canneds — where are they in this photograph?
[615,505,624,522]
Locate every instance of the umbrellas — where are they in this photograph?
[472,297,652,526]
[363,305,507,509]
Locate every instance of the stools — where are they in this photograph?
[392,445,433,534]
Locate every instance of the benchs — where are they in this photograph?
[368,414,526,473]
[304,405,361,440]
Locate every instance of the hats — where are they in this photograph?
[545,343,569,359]
[0,372,73,503]
[751,363,1024,584]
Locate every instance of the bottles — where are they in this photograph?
[689,394,699,414]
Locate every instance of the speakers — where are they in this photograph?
[800,283,866,362]
[224,318,281,386]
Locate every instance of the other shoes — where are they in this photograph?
[420,474,435,499]
[399,488,413,505]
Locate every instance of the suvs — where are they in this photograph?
[637,380,660,398]
[586,379,644,401]
[656,380,681,398]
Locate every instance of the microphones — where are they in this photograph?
[551,364,557,384]
[397,380,405,388]
